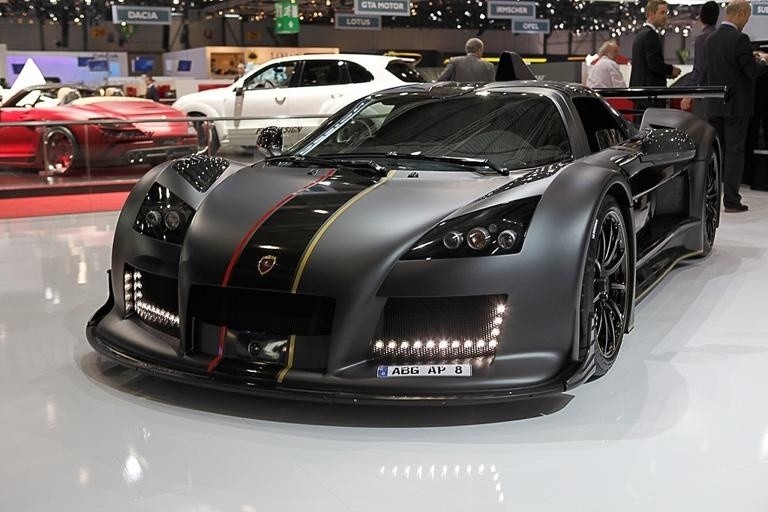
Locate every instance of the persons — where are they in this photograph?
[629,0,681,128]
[585,40,627,89]
[695,0,767,212]
[224,60,238,74]
[212,58,221,74]
[145,77,159,102]
[436,37,496,82]
[234,63,246,82]
[677,1,720,112]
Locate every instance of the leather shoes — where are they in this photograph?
[725,206,748,212]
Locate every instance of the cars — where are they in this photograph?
[172,54,435,155]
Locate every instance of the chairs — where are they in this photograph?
[35,84,125,108]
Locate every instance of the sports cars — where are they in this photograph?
[0,84,198,176]
[86,80,721,407]
